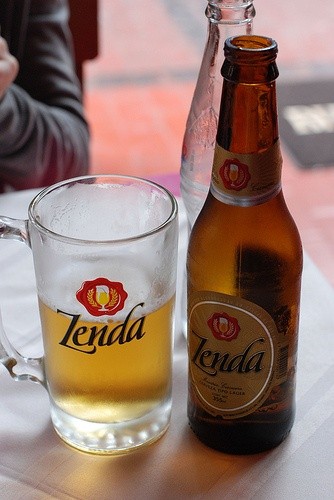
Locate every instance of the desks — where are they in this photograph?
[0,182,334,500]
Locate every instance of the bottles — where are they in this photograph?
[172,0,259,339]
[186,32,304,458]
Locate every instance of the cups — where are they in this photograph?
[0,173,179,457]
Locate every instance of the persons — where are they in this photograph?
[0,0,95,194]
